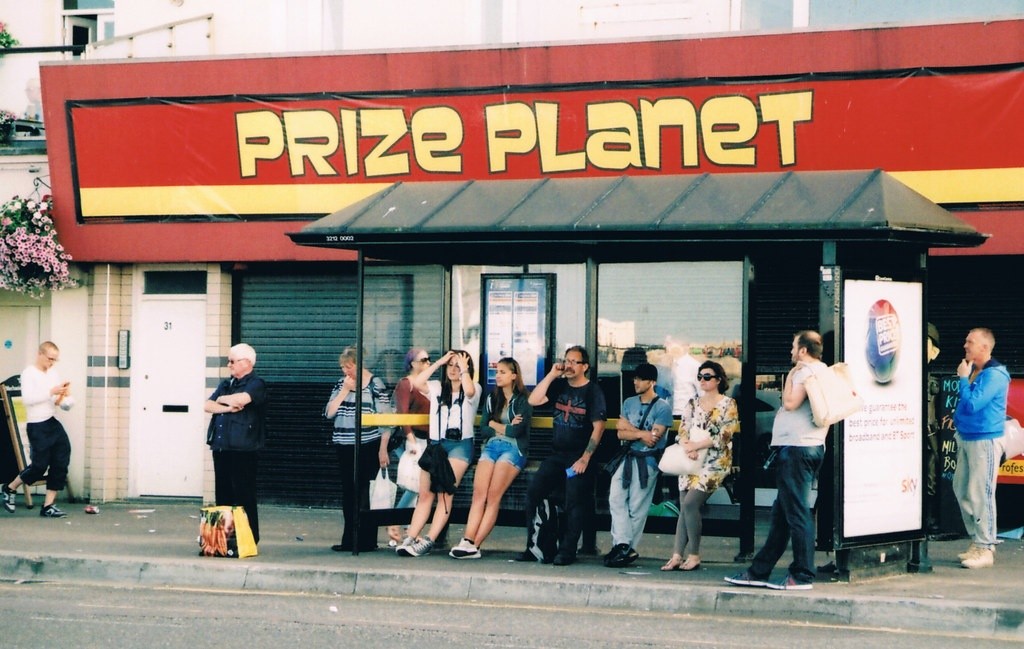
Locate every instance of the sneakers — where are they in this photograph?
[722,572,813,589]
[1,483,18,513]
[449,537,481,559]
[599,543,638,568]
[957,543,994,568]
[396,536,433,557]
[40,502,68,518]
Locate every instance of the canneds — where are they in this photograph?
[84,505,99,513]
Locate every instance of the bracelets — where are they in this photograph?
[460,370,469,375]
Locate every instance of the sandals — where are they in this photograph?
[660,554,700,571]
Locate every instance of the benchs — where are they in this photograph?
[466,459,741,504]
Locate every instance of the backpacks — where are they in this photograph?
[527,495,561,564]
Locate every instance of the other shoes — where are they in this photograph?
[331,543,378,552]
[386,526,421,543]
[515,548,577,566]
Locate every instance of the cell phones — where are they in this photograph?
[565,467,577,478]
[64,382,71,388]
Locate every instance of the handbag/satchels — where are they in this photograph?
[603,443,631,476]
[198,504,258,558]
[397,440,424,493]
[368,464,397,509]
[657,426,711,476]
[802,361,865,427]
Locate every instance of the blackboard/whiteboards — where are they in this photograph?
[939,375,966,505]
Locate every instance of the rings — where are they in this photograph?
[652,432,655,435]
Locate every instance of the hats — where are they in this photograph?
[404,348,421,372]
[633,363,657,382]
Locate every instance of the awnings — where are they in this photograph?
[928,210,1024,256]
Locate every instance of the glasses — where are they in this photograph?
[42,352,60,363]
[414,356,431,362]
[697,372,719,381]
[563,359,585,365]
[227,358,246,364]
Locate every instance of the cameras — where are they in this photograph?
[445,428,462,440]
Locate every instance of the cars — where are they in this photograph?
[598,364,782,489]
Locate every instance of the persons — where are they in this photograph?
[516,345,608,566]
[724,330,829,590]
[661,360,741,571]
[952,327,1011,570]
[449,357,533,560]
[2,342,71,518]
[325,344,482,557]
[927,323,959,542]
[204,343,267,545]
[603,363,673,568]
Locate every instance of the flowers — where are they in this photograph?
[0,194,76,299]
